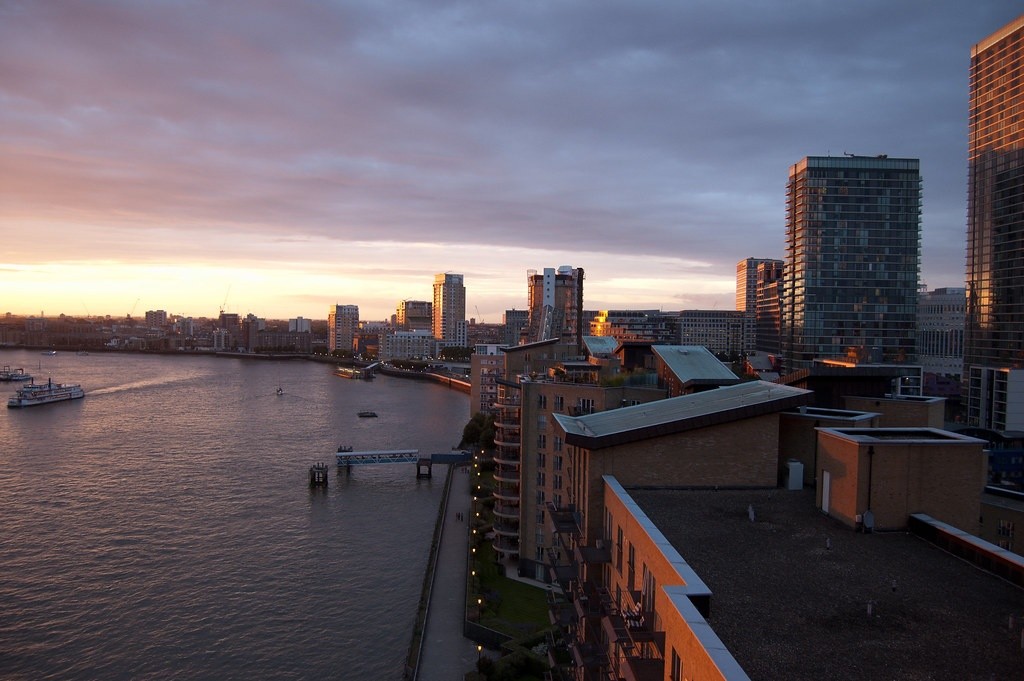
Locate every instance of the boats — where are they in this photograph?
[77,351,88,356]
[276,389,283,395]
[0,365,34,381]
[41,351,56,355]
[333,364,376,379]
[6,377,84,408]
[356,409,379,418]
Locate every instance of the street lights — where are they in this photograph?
[476,512,479,534]
[474,496,477,516]
[313,351,471,373]
[479,598,482,625]
[471,569,476,594]
[475,456,477,464]
[475,464,477,474]
[477,472,480,486]
[481,450,484,463]
[472,548,476,568]
[477,486,481,498]
[477,645,483,673]
[472,529,476,548]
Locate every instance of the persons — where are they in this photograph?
[464,466,469,474]
[456,512,464,522]
